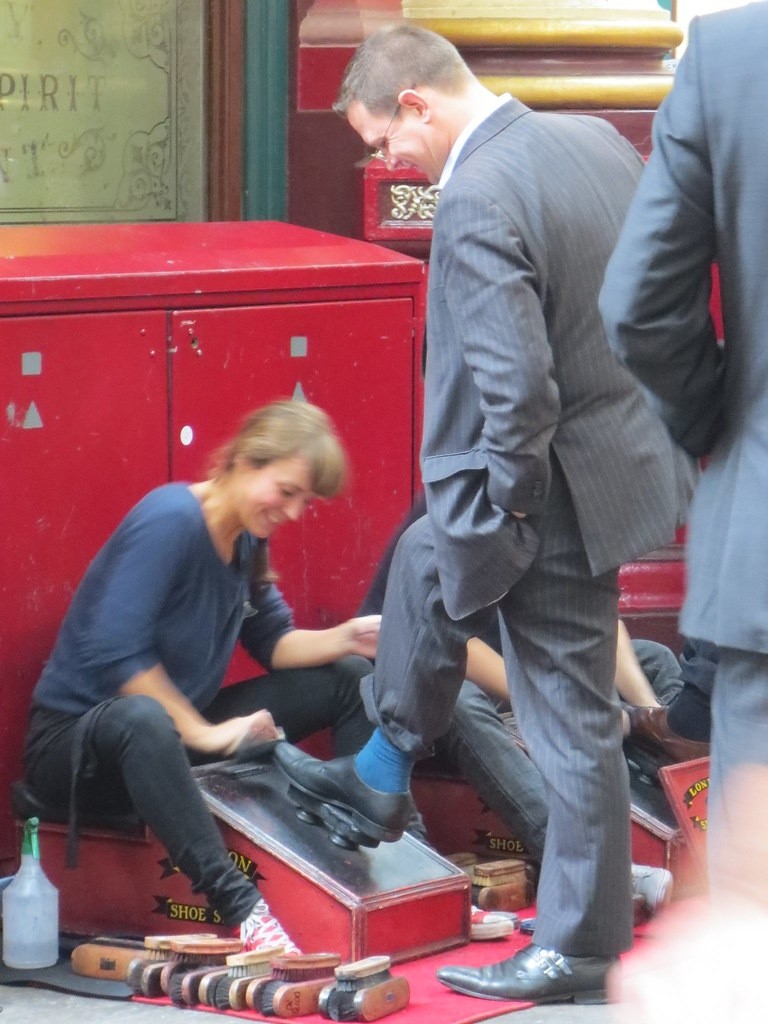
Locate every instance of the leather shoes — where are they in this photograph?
[625,706,710,763]
[275,739,410,843]
[436,943,626,1006]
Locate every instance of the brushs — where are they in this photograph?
[448,851,537,910]
[70,932,412,1023]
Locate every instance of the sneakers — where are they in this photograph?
[631,862,673,913]
[234,914,302,958]
[472,906,514,940]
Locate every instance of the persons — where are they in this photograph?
[596,0,768,890]
[270,26,718,1006]
[12,399,682,955]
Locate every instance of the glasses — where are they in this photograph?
[369,83,420,168]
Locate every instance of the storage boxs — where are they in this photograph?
[0,223,429,880]
